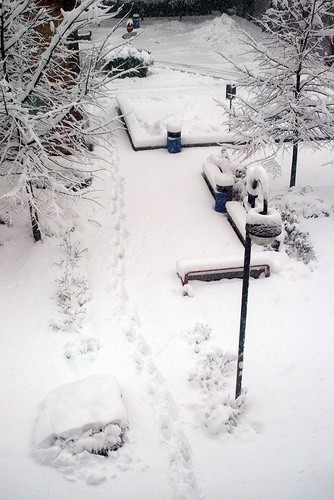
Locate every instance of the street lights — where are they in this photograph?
[235,165,281,400]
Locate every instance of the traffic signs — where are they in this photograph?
[226,84,236,100]
[126,23,133,33]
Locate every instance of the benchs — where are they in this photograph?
[203,162,223,199]
[226,201,253,247]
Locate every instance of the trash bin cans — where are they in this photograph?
[214,185,234,214]
[166,130,182,154]
[132,14,140,28]
